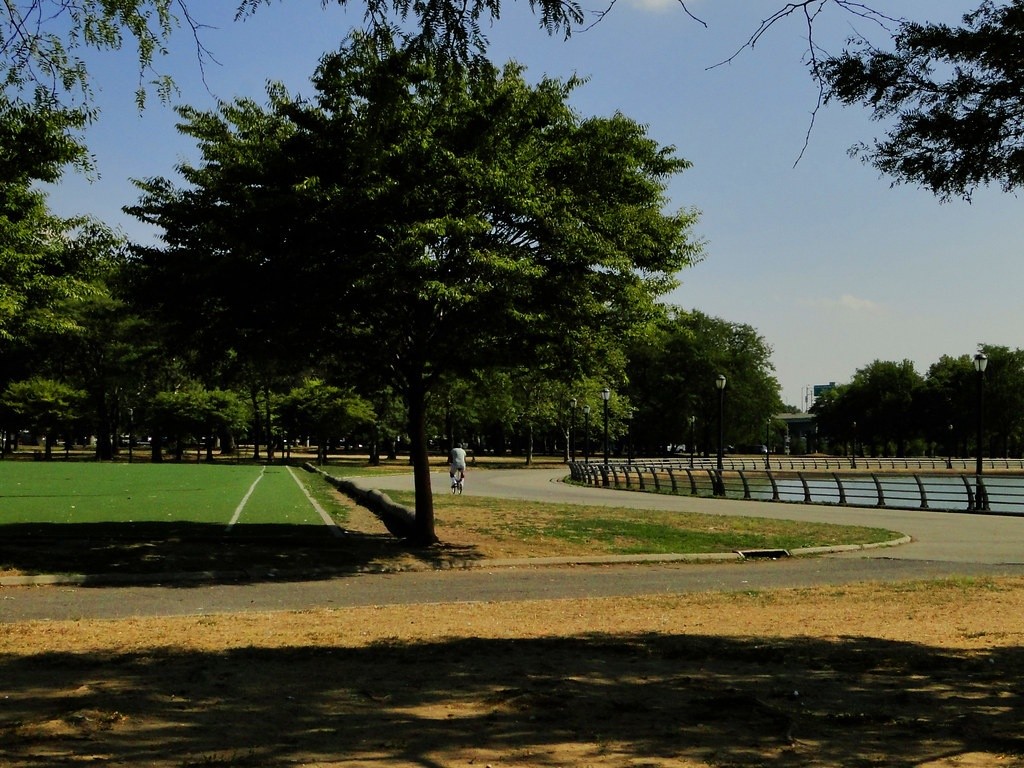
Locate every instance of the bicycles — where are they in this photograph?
[449,463,462,494]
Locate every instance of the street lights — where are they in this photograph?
[948,425,953,468]
[583,404,589,463]
[852,421,856,467]
[973,353,987,509]
[601,387,610,474]
[715,374,726,476]
[570,398,577,462]
[689,416,695,468]
[766,418,771,468]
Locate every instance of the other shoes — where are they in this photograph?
[451,483,457,488]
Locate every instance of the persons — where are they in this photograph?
[447,442,467,487]
